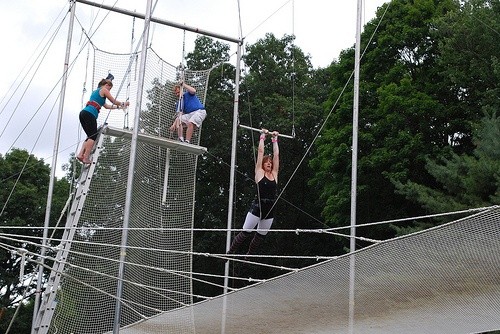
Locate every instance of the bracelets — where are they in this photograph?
[117,105,119,109]
[272,139,278,142]
[122,102,124,106]
[260,136,266,139]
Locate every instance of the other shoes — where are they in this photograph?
[179,138,184,142]
[185,140,189,143]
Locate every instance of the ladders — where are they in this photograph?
[33,123,108,334]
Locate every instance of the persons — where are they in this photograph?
[170,80,207,143]
[78,79,129,164]
[223,129,280,263]
